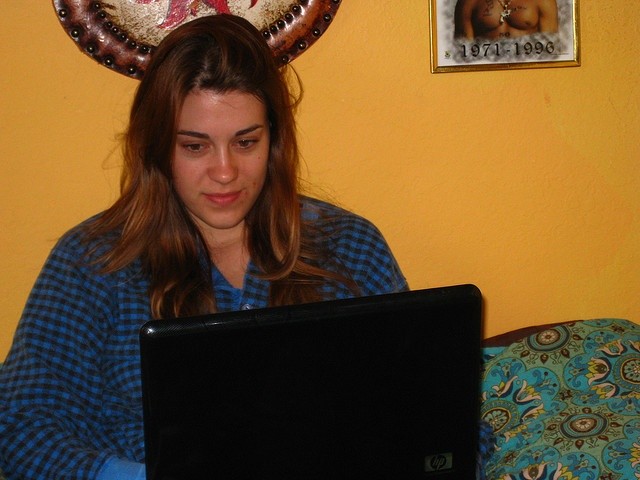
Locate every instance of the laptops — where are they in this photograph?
[139,284,484,480]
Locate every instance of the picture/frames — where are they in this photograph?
[428,0,581,77]
[51,0,341,83]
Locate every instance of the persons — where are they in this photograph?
[0,13,413,479]
[453,0,562,43]
